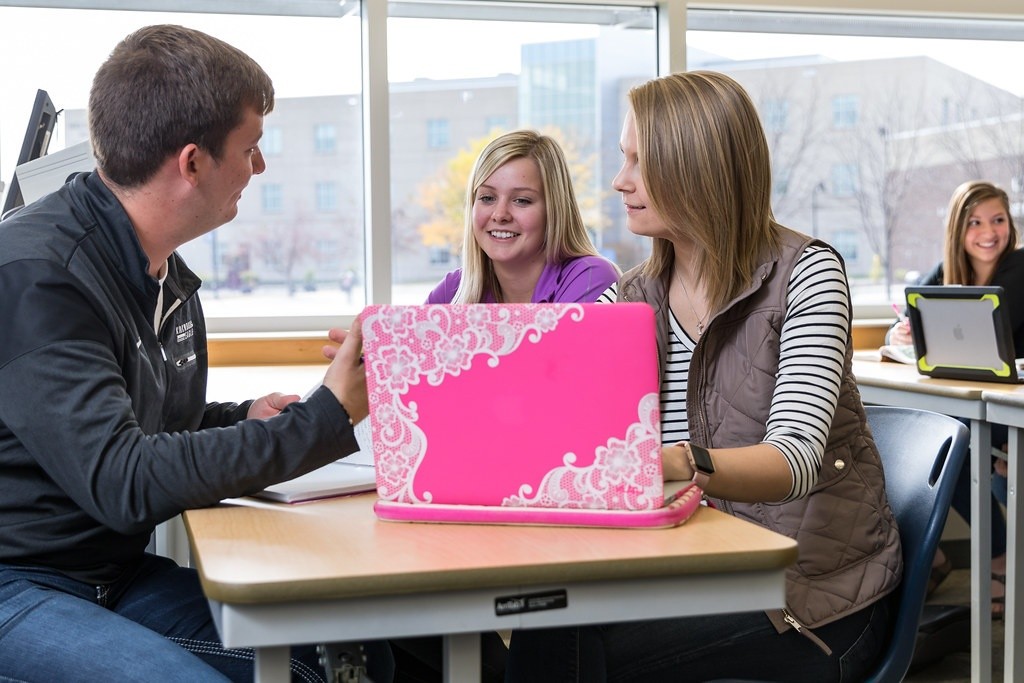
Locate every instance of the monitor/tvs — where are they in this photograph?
[2,88,58,223]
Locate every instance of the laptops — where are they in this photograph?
[904,286,1024,384]
[361,302,705,530]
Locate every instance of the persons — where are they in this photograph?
[1,24,370,683]
[505,68,905,683]
[420,130,624,304]
[885,180,1024,618]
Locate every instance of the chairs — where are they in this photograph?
[865,406,970,683]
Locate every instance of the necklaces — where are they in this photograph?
[673,263,710,337]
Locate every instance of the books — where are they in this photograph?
[852,344,916,366]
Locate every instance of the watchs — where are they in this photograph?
[673,441,715,489]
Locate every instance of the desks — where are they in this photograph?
[851,359,1024,683]
[154,487,800,683]
